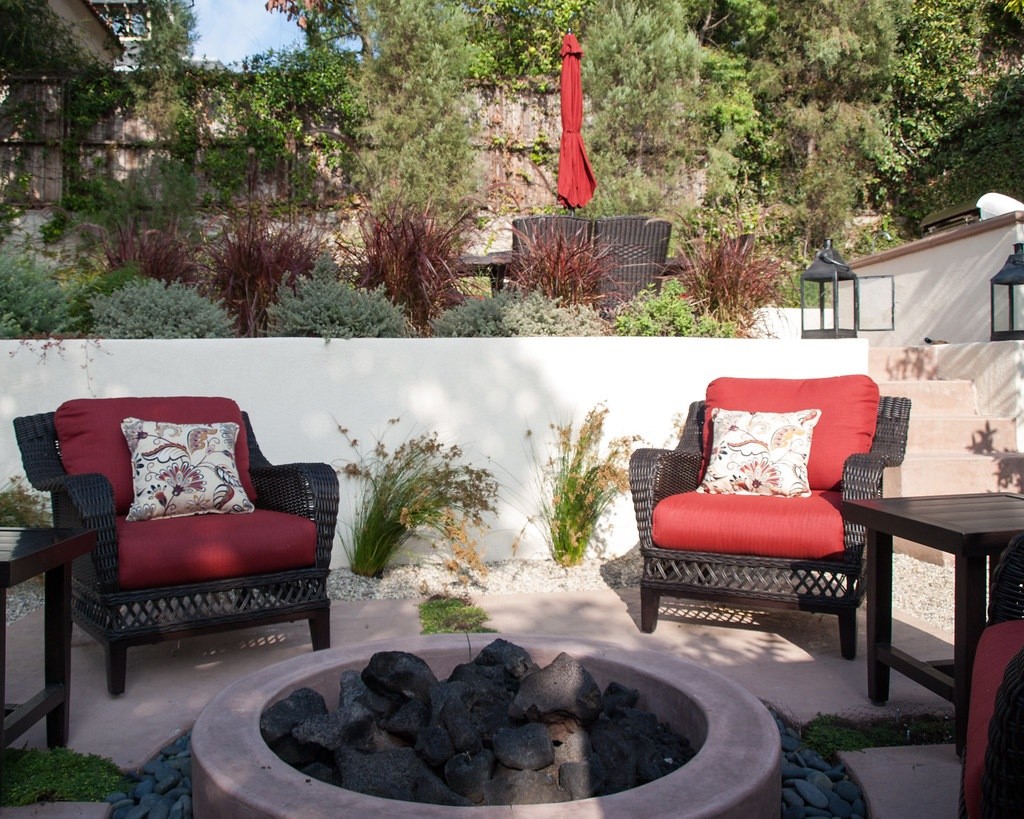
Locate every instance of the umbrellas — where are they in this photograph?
[557,27,598,217]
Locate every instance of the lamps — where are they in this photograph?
[989,243,1024,339]
[800,239,896,339]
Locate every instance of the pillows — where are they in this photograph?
[697,407,823,497]
[120,417,254,523]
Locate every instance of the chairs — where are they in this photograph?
[711,233,757,263]
[958,533,1024,819]
[629,376,912,659]
[12,395,340,698]
[508,217,671,319]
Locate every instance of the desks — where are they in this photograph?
[444,250,689,298]
[0,526,98,754]
[839,494,1024,761]
